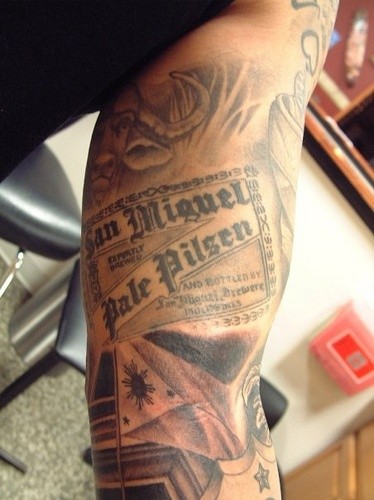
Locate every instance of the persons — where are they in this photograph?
[0,0,343,500]
